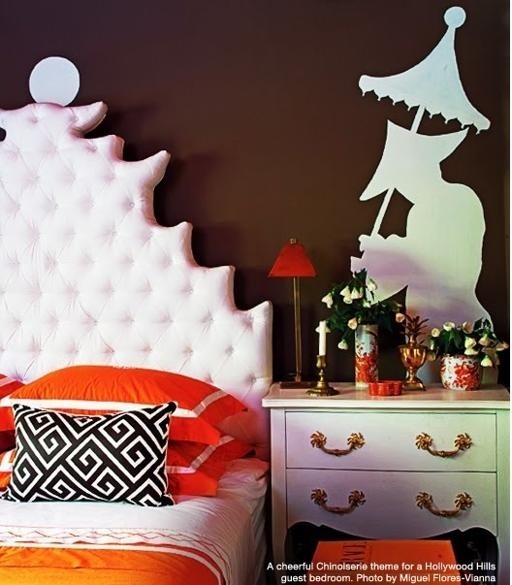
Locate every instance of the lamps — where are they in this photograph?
[267,238,319,389]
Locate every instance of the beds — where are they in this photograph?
[0,102,273,584]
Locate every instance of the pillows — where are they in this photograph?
[0,365,253,507]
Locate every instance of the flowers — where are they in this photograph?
[425,316,509,368]
[315,267,406,349]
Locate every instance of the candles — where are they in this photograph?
[319,320,326,356]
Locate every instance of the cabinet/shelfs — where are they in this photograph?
[261,382,510,585]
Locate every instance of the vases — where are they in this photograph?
[439,355,482,390]
[355,325,379,390]
